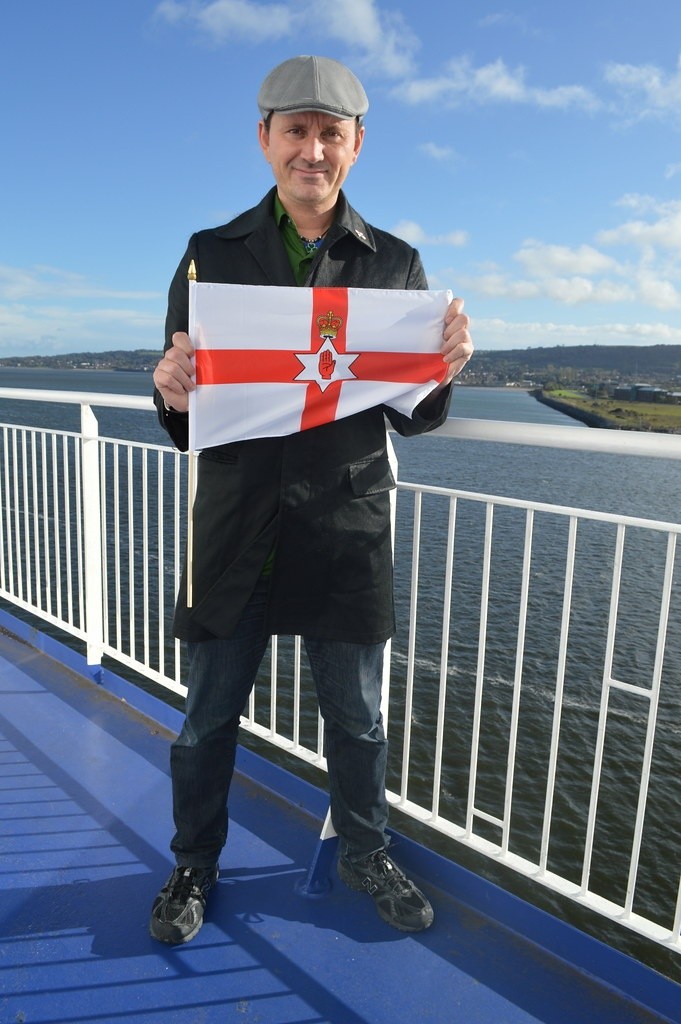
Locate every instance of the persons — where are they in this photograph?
[151,54,473,943]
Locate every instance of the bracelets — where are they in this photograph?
[163,401,187,414]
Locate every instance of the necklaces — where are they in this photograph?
[301,227,331,254]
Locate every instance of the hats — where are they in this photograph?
[258,55,368,124]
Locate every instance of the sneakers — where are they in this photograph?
[337,842,434,932]
[149,863,220,944]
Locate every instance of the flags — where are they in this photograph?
[188,280,453,451]
